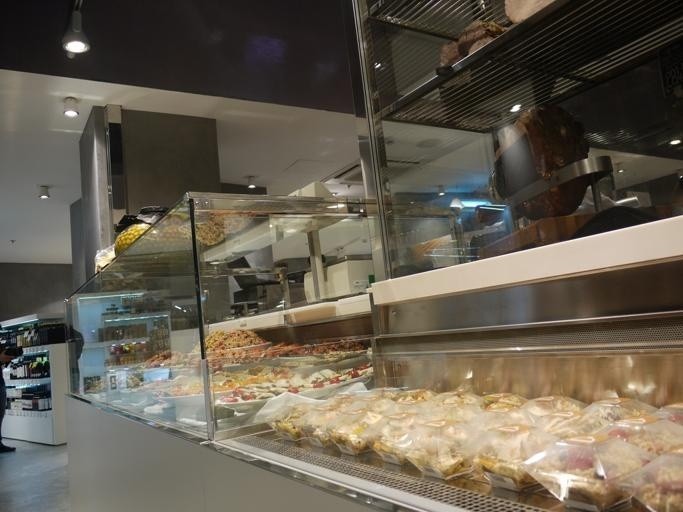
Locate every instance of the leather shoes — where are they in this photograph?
[0,442,16,452]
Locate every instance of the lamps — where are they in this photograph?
[62,11,89,53]
[62,99,79,117]
[248,176,255,188]
[38,188,49,200]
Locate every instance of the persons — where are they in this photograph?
[0,324,24,454]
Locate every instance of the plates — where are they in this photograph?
[218,368,373,412]
[276,350,368,363]
[188,342,271,358]
[160,390,233,406]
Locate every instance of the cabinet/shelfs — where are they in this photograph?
[66,192,634,511]
[351,0,683,406]
[0,312,70,446]
